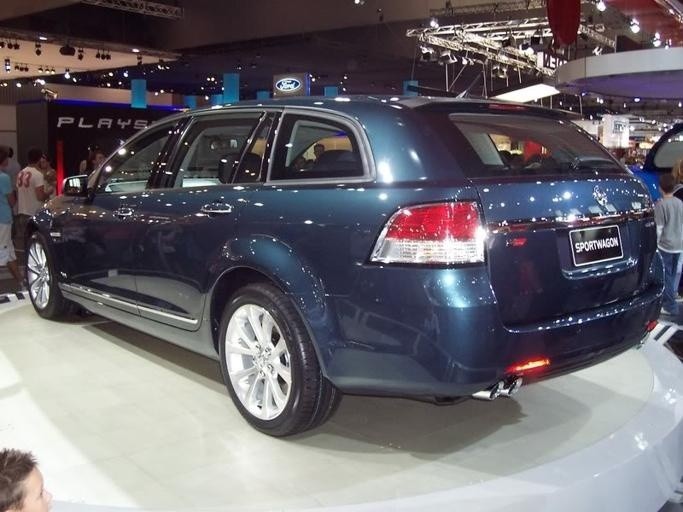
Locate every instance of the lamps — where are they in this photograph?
[1,29,324,133]
[336,0,682,134]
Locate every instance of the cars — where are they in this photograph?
[17,89,670,442]
[638,121,682,210]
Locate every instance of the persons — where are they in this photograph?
[0,144,103,294]
[671,160,682,202]
[654,173,683,315]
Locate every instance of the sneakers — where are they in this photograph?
[17,279,28,291]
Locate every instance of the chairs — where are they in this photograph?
[306,150,356,177]
[218,152,261,184]
